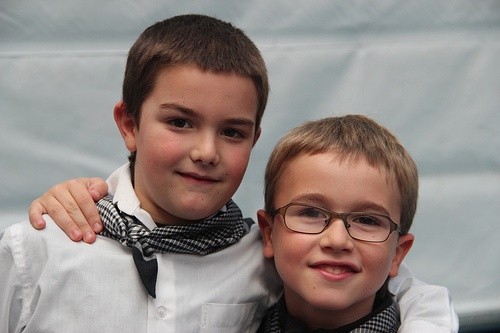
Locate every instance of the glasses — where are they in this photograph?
[265,202,403,242]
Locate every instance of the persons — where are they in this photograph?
[28,115,419,333]
[0,14,460,333]
